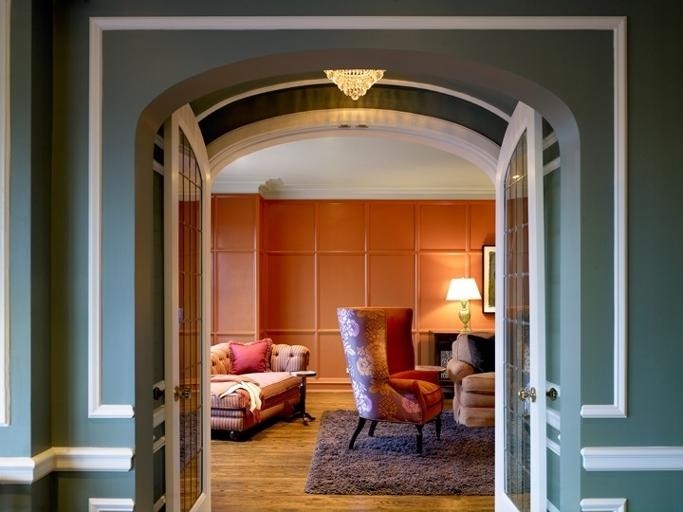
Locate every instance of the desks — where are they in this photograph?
[282,371,318,427]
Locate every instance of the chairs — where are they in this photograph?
[334,307,447,453]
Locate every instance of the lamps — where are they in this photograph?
[324,70,387,100]
[446,278,483,331]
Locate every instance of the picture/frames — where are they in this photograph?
[482,245,528,313]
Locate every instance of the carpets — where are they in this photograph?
[304,410,532,496]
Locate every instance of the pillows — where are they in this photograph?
[450,333,494,372]
[226,338,272,376]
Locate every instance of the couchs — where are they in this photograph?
[446,329,496,429]
[204,342,310,442]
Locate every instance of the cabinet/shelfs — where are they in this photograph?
[429,329,460,398]
